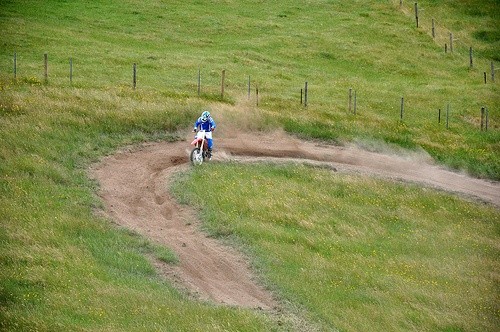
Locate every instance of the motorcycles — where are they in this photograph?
[190,130,212,166]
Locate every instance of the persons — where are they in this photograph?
[193,110,216,159]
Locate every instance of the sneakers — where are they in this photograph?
[209,154,213,160]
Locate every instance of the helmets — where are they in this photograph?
[202,111,210,120]
